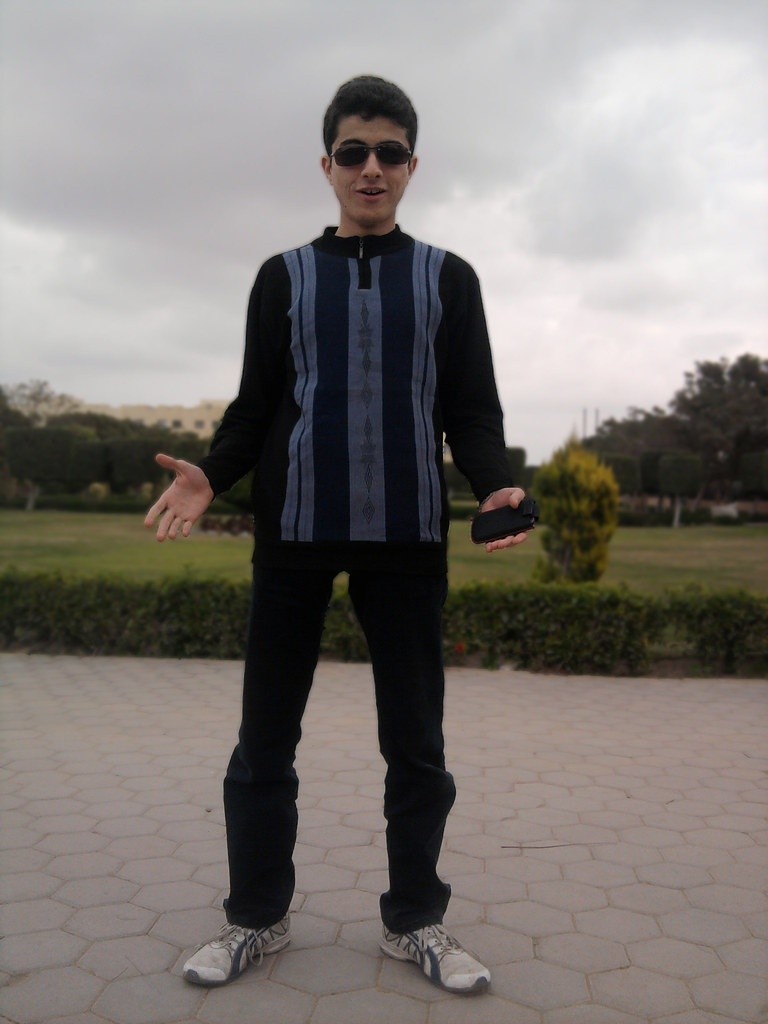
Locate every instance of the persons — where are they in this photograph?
[144,74,530,993]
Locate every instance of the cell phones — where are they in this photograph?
[470,500,538,544]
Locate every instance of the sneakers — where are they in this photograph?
[182,913,291,985]
[380,922,491,994]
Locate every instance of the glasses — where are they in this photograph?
[330,143,411,167]
